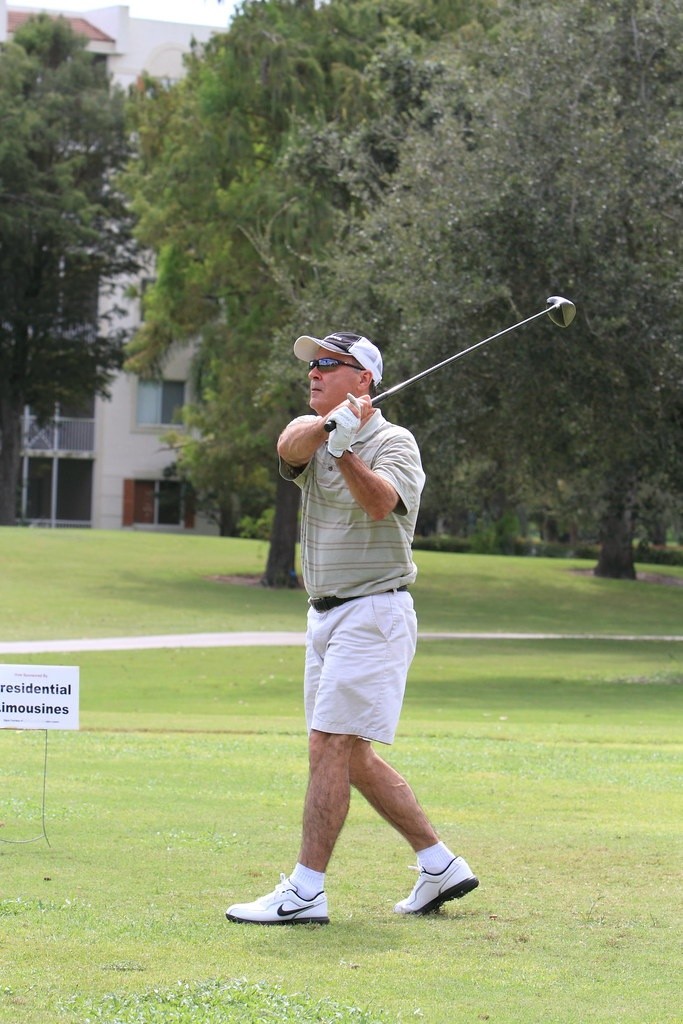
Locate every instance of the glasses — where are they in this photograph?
[308,357,365,371]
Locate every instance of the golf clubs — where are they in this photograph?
[322,295,578,433]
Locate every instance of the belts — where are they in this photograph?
[310,585,407,613]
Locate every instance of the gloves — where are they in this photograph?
[328,393,361,458]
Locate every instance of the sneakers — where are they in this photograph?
[226,873,329,925]
[394,858,479,914]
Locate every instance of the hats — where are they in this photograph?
[293,332,383,390]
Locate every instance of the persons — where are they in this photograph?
[226,332,478,926]
